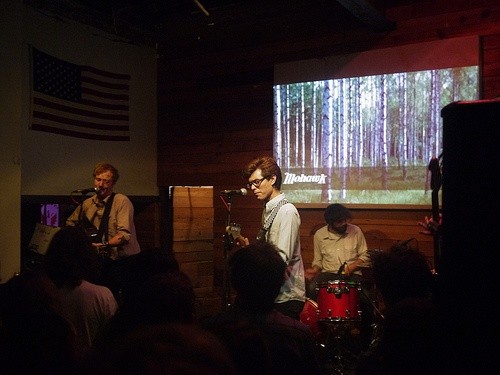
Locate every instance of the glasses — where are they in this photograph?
[247,176,268,189]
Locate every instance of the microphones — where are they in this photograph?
[72,187,100,194]
[401,238,415,246]
[219,188,247,195]
[338,262,346,274]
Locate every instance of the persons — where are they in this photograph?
[0,225,434,375]
[66,162,141,260]
[418,214,442,235]
[305,204,374,295]
[237,157,306,322]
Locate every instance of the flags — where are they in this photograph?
[27,43,132,141]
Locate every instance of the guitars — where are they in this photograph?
[426,153,447,270]
[75,217,111,256]
[224,225,251,248]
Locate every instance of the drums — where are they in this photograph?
[315,280,361,325]
